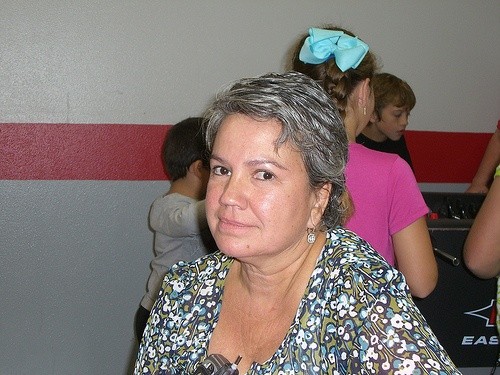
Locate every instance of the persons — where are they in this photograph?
[466,111,500,194]
[354,72,417,177]
[133,69,464,374]
[134,116,219,350]
[292,26,438,301]
[463,162,500,339]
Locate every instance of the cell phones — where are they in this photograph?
[193,353,242,375]
